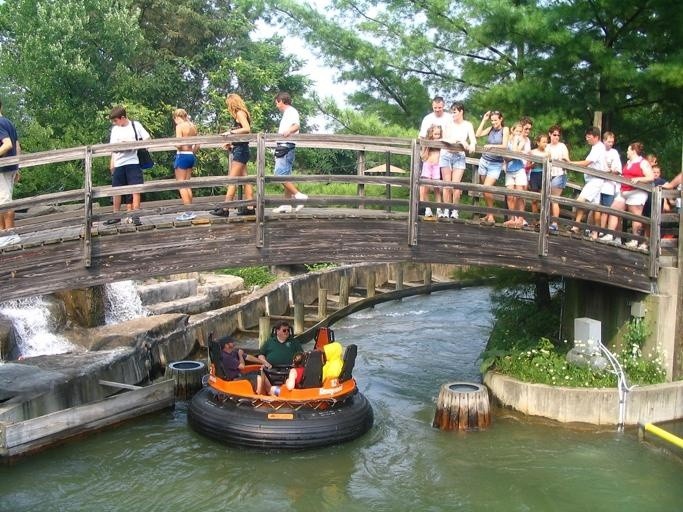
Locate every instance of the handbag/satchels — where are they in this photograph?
[131,120,154,168]
[274,146,295,158]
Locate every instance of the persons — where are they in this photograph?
[475,111,509,223]
[599,131,622,236]
[173,108,200,221]
[0,101,22,247]
[220,337,271,396]
[103,107,151,226]
[420,124,443,218]
[520,117,533,225]
[659,173,683,213]
[209,94,255,216]
[439,102,476,219]
[270,353,304,397]
[545,126,569,230]
[504,123,527,228]
[599,143,654,248]
[646,154,658,166]
[637,165,670,255]
[323,342,343,386]
[258,322,304,367]
[526,134,551,228]
[273,92,308,213]
[561,126,607,240]
[419,97,450,142]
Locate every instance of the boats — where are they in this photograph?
[186,372,374,453]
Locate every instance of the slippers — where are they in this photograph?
[481,216,488,221]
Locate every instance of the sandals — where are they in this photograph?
[549,224,558,230]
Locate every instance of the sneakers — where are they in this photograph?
[424,206,433,217]
[132,216,143,225]
[443,208,449,218]
[103,218,121,226]
[625,239,638,248]
[272,205,291,213]
[209,207,229,217]
[0,233,15,247]
[295,194,308,212]
[571,226,580,234]
[436,208,444,218]
[638,243,648,251]
[599,233,613,241]
[176,212,192,219]
[610,239,621,244]
[236,207,255,215]
[10,228,20,243]
[450,209,458,219]
[515,216,528,226]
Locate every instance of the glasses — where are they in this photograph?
[491,110,499,113]
[283,328,289,332]
[553,134,560,136]
